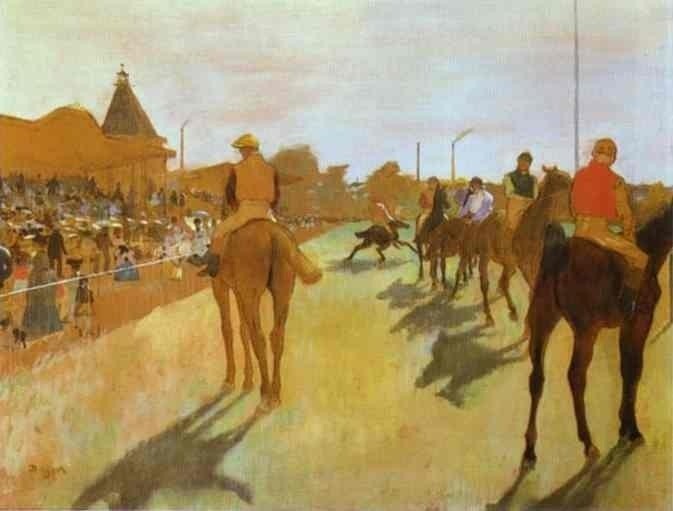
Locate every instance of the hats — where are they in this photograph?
[229,133,259,149]
[595,139,617,153]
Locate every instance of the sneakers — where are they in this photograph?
[187,252,221,266]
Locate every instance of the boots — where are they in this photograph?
[623,285,638,313]
